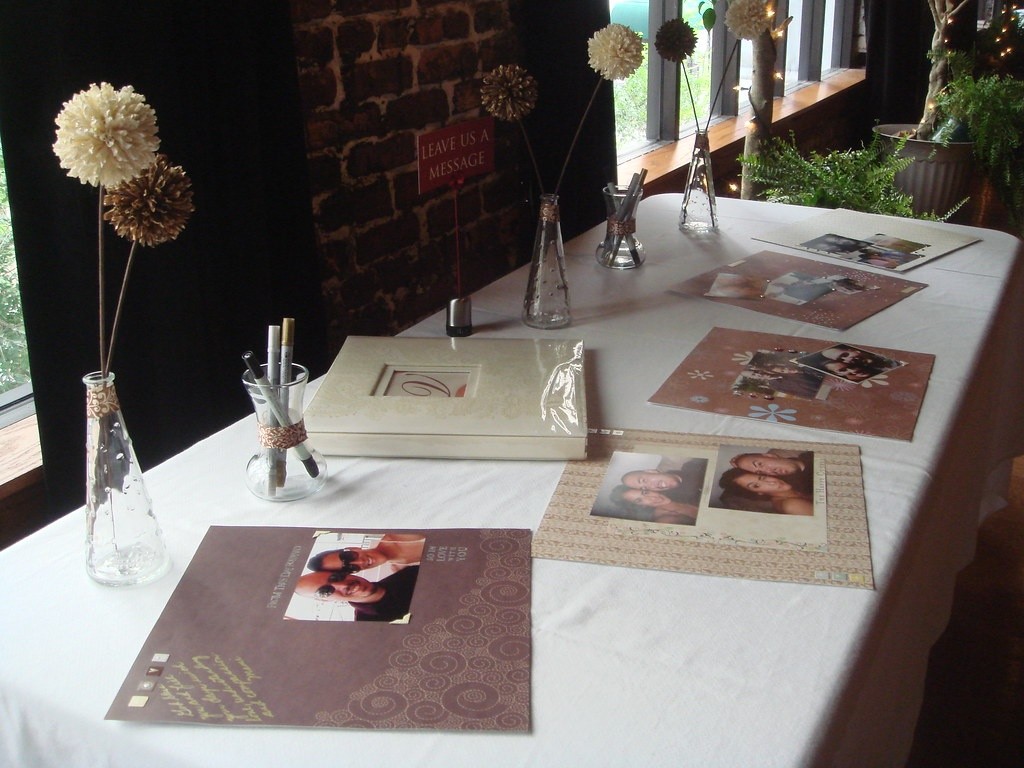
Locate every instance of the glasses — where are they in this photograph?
[340,550,361,573]
[316,573,346,597]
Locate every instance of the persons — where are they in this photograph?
[716,446,813,518]
[709,234,921,397]
[609,457,708,526]
[295,533,424,626]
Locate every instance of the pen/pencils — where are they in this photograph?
[239,316,320,498]
[600,167,649,269]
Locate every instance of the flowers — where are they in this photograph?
[655,0,769,234]
[52,82,192,587]
[479,25,642,331]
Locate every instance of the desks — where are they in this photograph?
[0,192,1024,768]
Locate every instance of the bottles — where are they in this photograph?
[446,296,473,337]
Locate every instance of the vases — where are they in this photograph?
[83,370,169,586]
[678,129,717,232]
[521,193,573,328]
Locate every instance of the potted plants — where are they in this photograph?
[872,0,976,223]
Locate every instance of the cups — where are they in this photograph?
[242,363,328,502]
[595,186,645,269]
